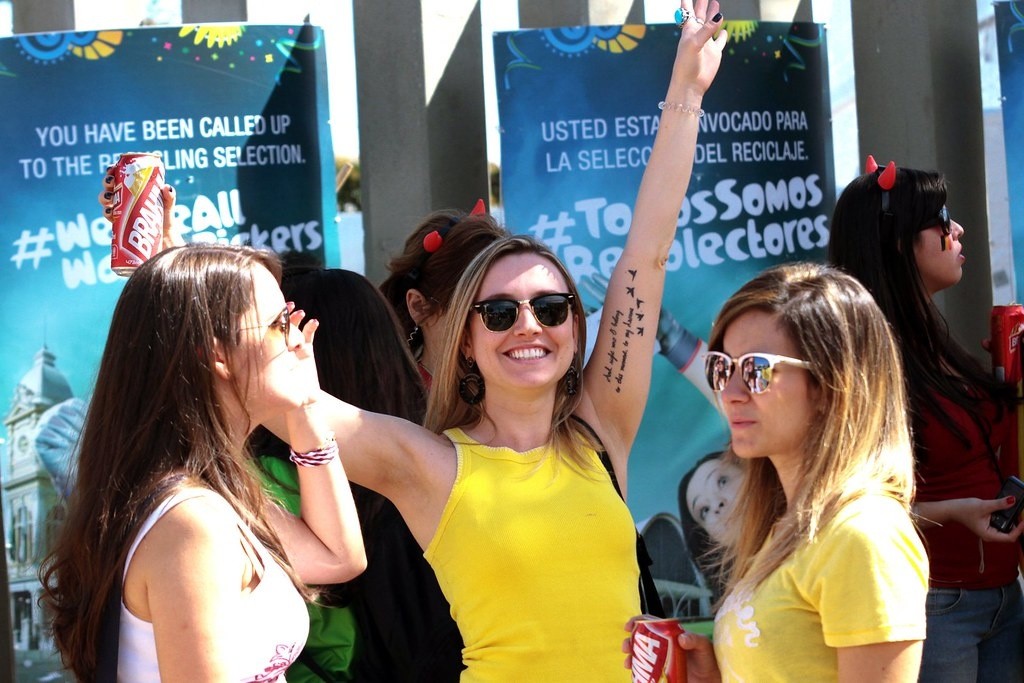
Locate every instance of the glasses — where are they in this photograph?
[919,203,951,235]
[700,350,815,396]
[470,291,574,333]
[237,306,291,338]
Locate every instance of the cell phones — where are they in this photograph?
[989,476,1024,532]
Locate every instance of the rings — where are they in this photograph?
[675,7,696,28]
[694,18,705,26]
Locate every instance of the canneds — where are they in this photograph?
[991,297,1024,382]
[111,154,166,277]
[631,618,688,683]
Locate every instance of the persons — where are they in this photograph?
[243,267,430,683]
[826,155,1023,683]
[617,259,933,683]
[33,242,368,683]
[95,1,731,683]
[574,267,751,616]
[375,201,514,393]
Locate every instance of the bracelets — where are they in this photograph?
[658,100,706,118]
[286,432,339,468]
